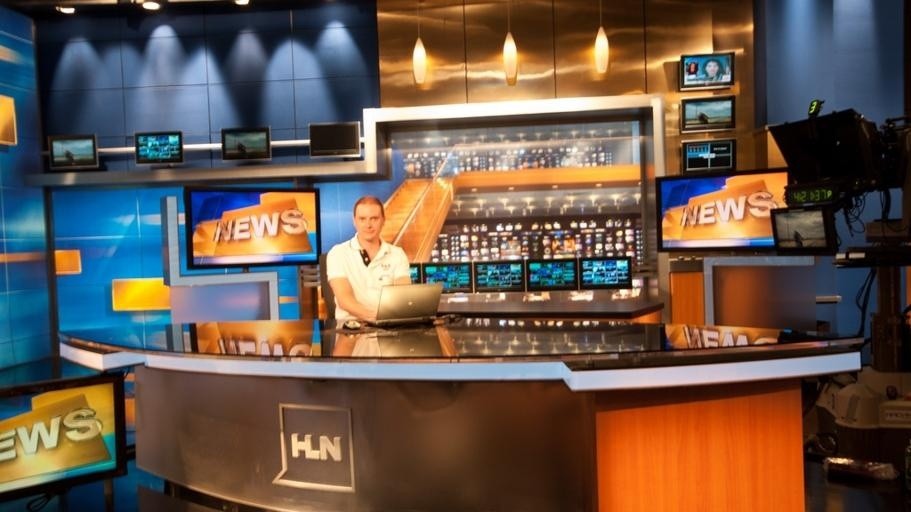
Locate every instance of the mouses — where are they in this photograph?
[344,320,361,330]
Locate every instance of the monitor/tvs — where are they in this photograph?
[221,126,272,166]
[134,131,184,168]
[679,52,734,91]
[770,202,837,256]
[0,371,127,505]
[45,133,99,170]
[183,186,321,269]
[680,94,736,134]
[681,138,736,176]
[308,121,361,159]
[655,166,793,252]
[409,255,632,294]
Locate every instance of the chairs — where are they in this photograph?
[319,255,335,319]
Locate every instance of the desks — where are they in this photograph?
[59,319,865,512]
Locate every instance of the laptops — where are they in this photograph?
[360,283,442,326]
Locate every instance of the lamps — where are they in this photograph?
[595,0,609,74]
[503,0,518,85]
[412,0,427,84]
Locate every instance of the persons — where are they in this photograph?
[697,59,730,81]
[326,195,412,329]
[331,321,381,358]
